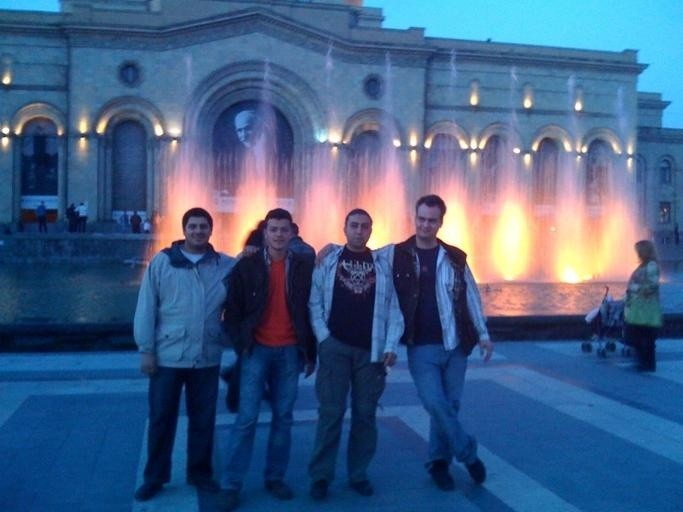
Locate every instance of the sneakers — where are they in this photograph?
[355,480,372,495]
[311,481,326,498]
[470,458,485,483]
[431,460,452,489]
[198,483,239,512]
[135,483,162,501]
[266,480,291,499]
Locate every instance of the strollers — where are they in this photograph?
[580,287,633,358]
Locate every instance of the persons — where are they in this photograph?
[289,223,317,265]
[214,209,315,512]
[315,194,493,491]
[624,240,663,373]
[64,200,88,234]
[36,200,48,233]
[119,209,152,234]
[308,209,405,501]
[234,110,257,149]
[221,220,262,413]
[133,207,261,500]
